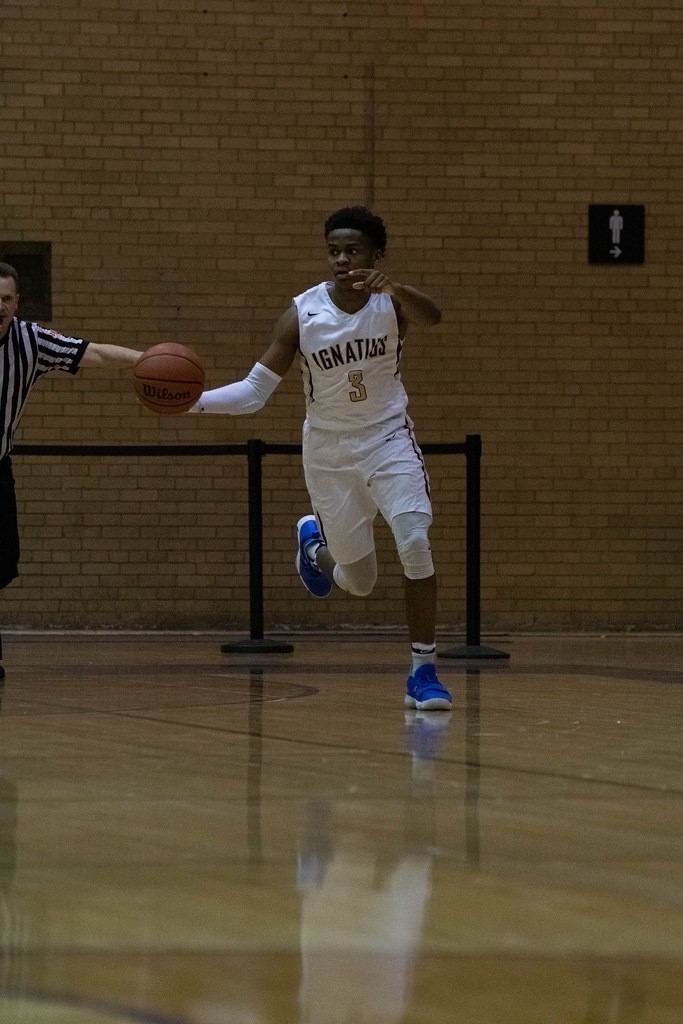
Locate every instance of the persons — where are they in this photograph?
[0,262,144,678]
[185,205,454,710]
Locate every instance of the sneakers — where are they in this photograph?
[404,709,453,751]
[405,662,453,711]
[295,514,334,598]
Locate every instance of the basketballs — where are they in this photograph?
[133,343,205,416]
